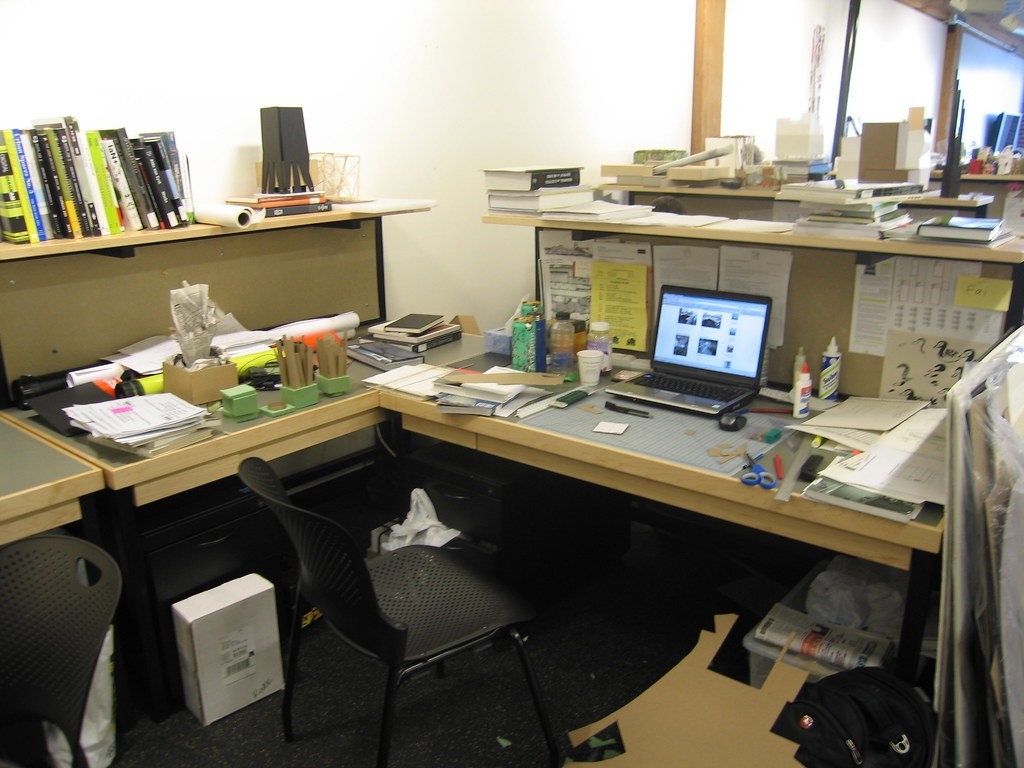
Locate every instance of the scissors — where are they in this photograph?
[740,453,777,489]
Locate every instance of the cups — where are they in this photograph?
[969,159,983,174]
[577,350,603,386]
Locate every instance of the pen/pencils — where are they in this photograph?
[811,435,824,448]
[750,408,793,414]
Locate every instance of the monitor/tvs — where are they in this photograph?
[992,111,1023,153]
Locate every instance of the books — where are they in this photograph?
[88,415,225,460]
[481,165,657,223]
[801,450,926,526]
[0,113,198,246]
[223,190,333,219]
[345,313,463,372]
[772,180,1017,249]
[601,135,755,187]
[359,362,528,419]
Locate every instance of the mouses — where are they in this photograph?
[719,414,746,430]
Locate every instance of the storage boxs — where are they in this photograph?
[777,134,823,156]
[171,573,285,726]
[601,164,735,188]
[742,558,841,689]
[163,360,242,405]
[705,136,754,169]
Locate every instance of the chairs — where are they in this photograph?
[238,456,563,768]
[0,535,122,768]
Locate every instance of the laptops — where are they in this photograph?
[604,284,773,417]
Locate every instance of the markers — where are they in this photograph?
[775,455,783,480]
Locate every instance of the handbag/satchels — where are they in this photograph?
[44,623,117,768]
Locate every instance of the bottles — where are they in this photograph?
[587,322,613,372]
[792,363,812,418]
[818,336,842,402]
[550,311,575,374]
[513,300,546,373]
[793,347,806,387]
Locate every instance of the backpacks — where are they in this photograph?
[771,667,938,768]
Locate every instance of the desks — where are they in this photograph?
[0,170,1024,684]
